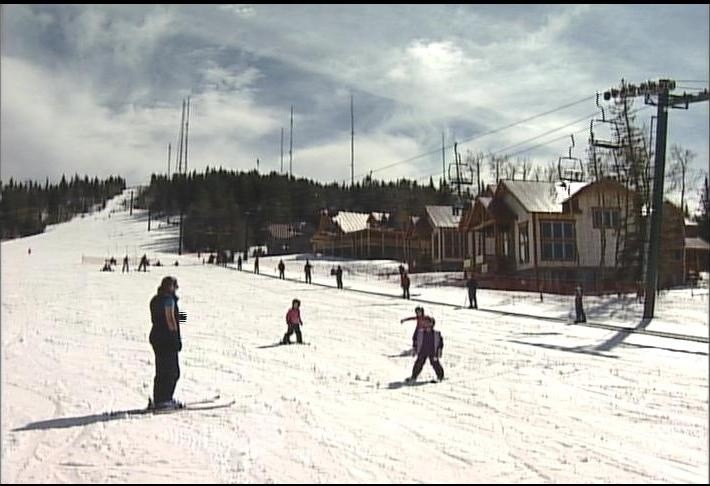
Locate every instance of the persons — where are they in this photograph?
[282,297,304,344]
[304,260,312,284]
[405,314,444,382]
[401,272,411,300]
[102,246,266,275]
[336,263,343,289]
[400,306,426,353]
[465,272,479,308]
[574,283,586,323]
[148,276,187,409]
[278,258,285,280]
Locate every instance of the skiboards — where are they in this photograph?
[388,349,419,357]
[389,376,450,389]
[105,395,236,417]
[258,340,311,348]
